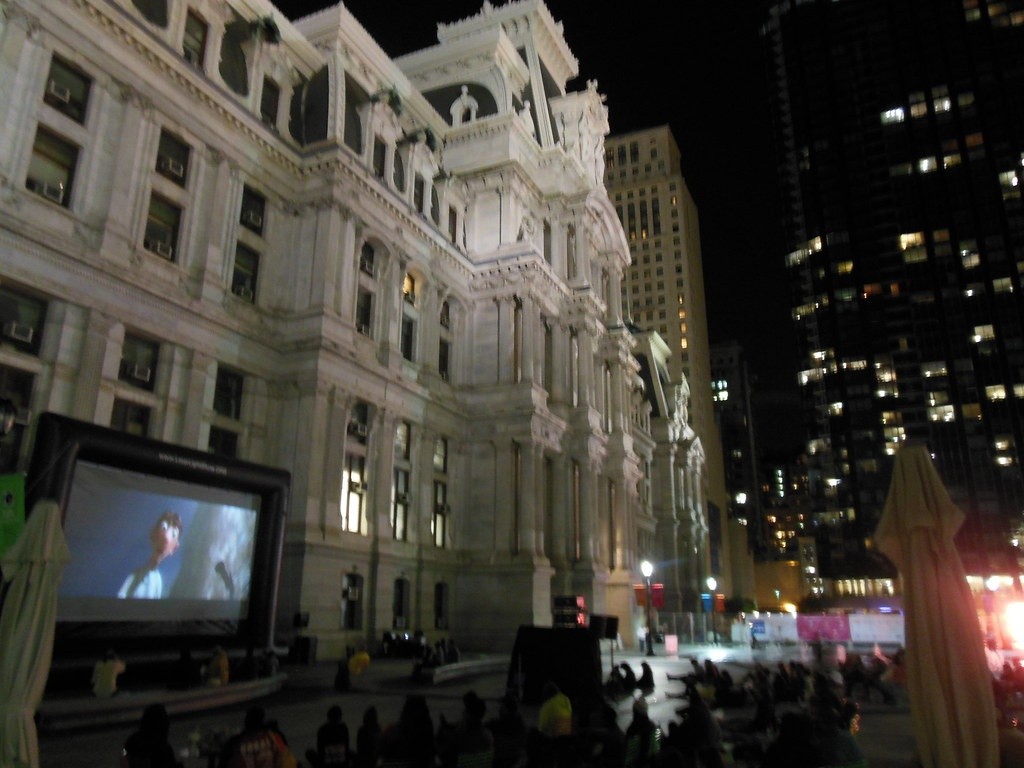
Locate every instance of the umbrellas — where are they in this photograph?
[2,498,70,767]
[869,445,1002,768]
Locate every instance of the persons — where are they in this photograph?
[90,629,1024,768]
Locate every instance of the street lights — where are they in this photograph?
[706,577,718,647]
[772,589,781,642]
[639,560,654,656]
